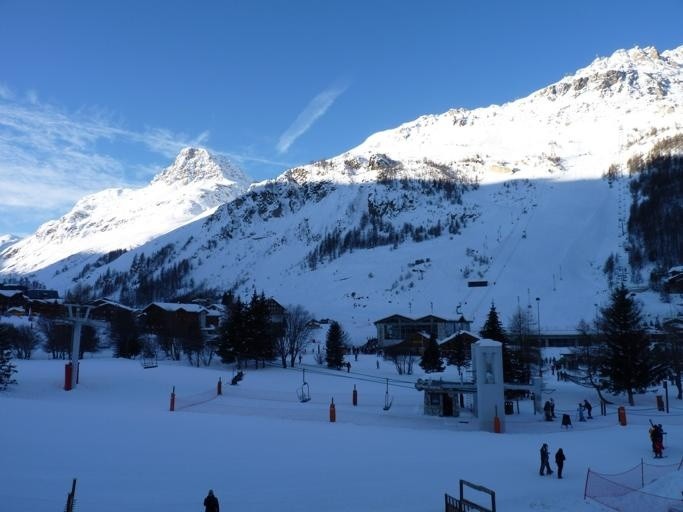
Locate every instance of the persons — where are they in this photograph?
[556,448,565,478]
[650,424,663,458]
[347,362,351,372]
[204,490,219,512]
[540,357,592,422]
[540,444,553,475]
[299,354,303,362]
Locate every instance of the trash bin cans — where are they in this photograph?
[504,401,513,415]
